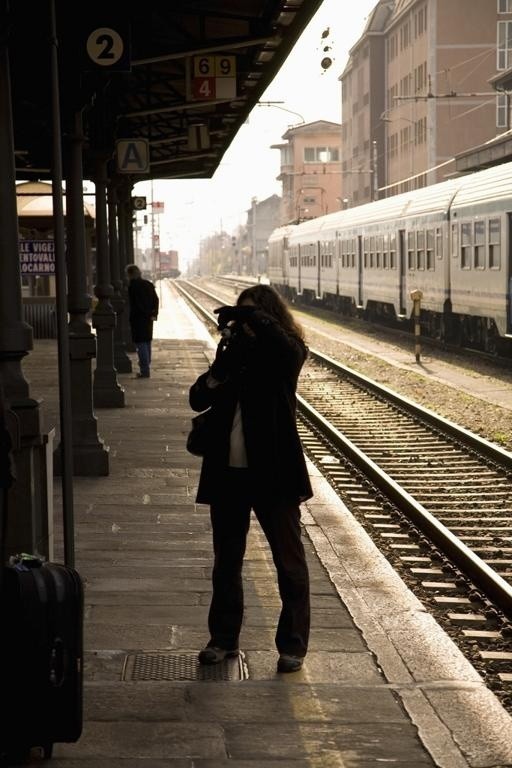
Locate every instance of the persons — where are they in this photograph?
[186,282,315,672]
[125,262,160,378]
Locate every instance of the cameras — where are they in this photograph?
[220,326,242,347]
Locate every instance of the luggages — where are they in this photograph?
[0,553,83,756]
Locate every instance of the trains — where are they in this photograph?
[266,158,511,358]
[157,249,182,278]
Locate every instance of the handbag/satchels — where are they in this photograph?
[186,410,212,457]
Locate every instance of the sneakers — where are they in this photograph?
[199,646,240,665]
[277,653,303,673]
